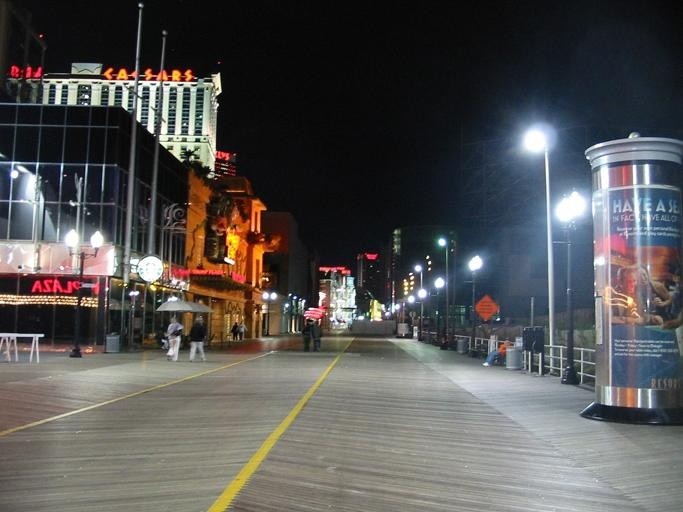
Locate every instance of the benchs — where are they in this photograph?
[0,331,45,363]
[417,331,506,366]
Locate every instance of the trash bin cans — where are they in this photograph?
[457,340,465,354]
[506,346,523,370]
[424,334,432,344]
[105,335,121,353]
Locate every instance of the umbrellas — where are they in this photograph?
[179,302,214,324]
[157,299,192,323]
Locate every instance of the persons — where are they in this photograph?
[481,336,512,367]
[229,322,238,341]
[301,322,311,352]
[600,260,683,331]
[158,325,168,350]
[164,317,183,362]
[235,320,248,341]
[309,320,323,352]
[186,317,205,361]
[194,314,209,352]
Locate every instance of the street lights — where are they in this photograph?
[521,125,555,377]
[282,291,306,336]
[381,263,429,342]
[553,189,591,386]
[262,291,278,336]
[466,253,484,358]
[436,237,452,349]
[61,226,107,358]
[431,275,445,346]
